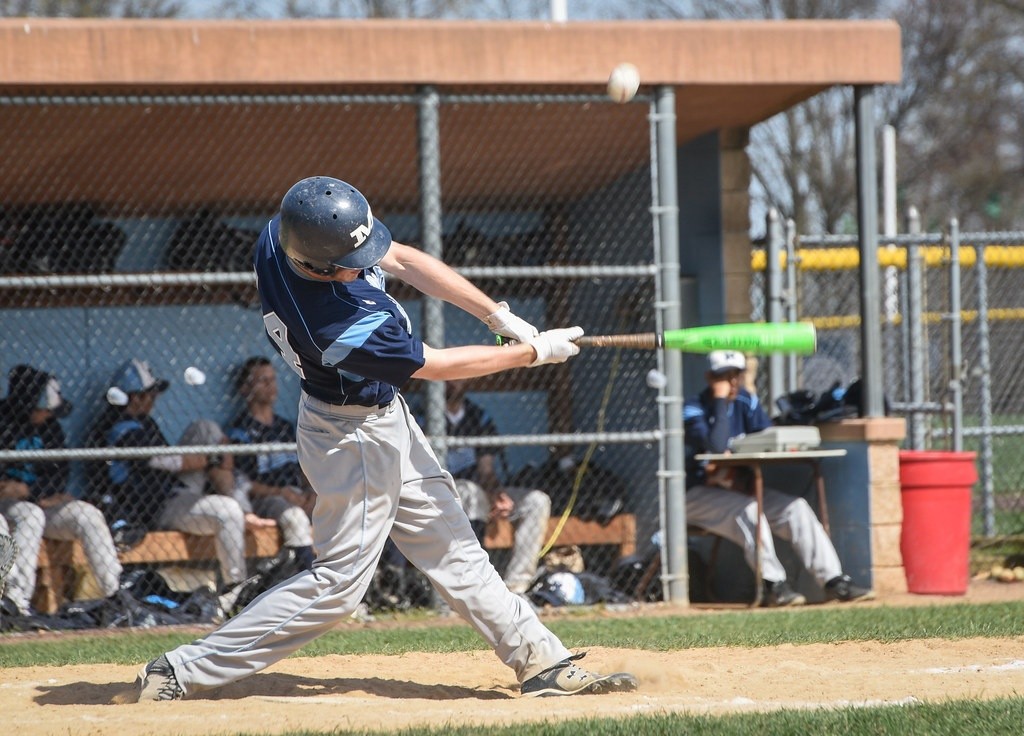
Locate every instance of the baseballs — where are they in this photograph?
[607,61,641,106]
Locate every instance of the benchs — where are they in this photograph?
[33,514,636,615]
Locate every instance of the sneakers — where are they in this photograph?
[137,659,182,703]
[520,651,636,696]
[766,581,807,606]
[826,574,875,604]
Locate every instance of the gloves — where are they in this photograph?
[520,325,583,368]
[484,300,536,345]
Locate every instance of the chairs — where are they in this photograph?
[633,527,722,603]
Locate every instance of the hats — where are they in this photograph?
[109,358,168,395]
[536,572,585,605]
[35,374,71,419]
[704,350,745,374]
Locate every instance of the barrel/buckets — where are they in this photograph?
[901,450,978,595]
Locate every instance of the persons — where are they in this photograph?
[0,356,551,631]
[138,175,638,701]
[683,350,877,606]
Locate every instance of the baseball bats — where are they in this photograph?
[494,319,818,358]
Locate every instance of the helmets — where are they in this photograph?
[279,175,392,274]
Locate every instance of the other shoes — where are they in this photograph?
[100,597,155,630]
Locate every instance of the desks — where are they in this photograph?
[694,449,848,609]
